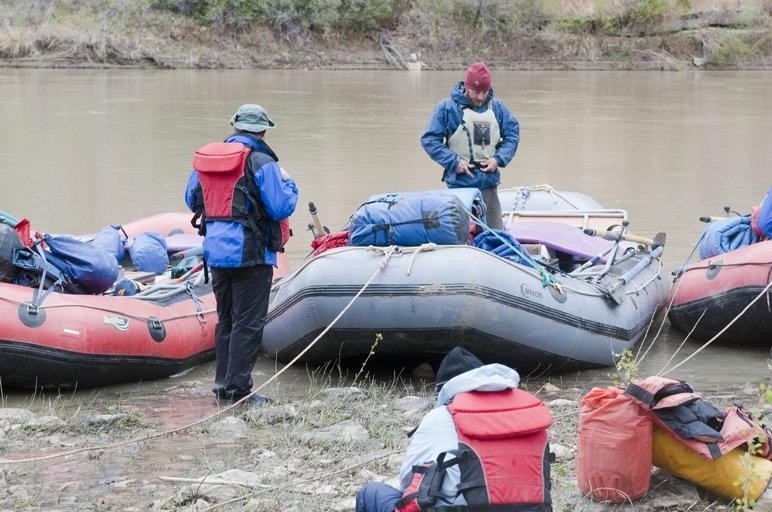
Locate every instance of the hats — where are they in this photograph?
[434,346,484,401]
[464,63,492,90]
[230,102,275,132]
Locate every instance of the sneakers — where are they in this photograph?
[216,388,273,406]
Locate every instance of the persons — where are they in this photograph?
[355,345,556,512]
[418,62,521,232]
[184,101,300,406]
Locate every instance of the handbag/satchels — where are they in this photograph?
[43,224,171,292]
